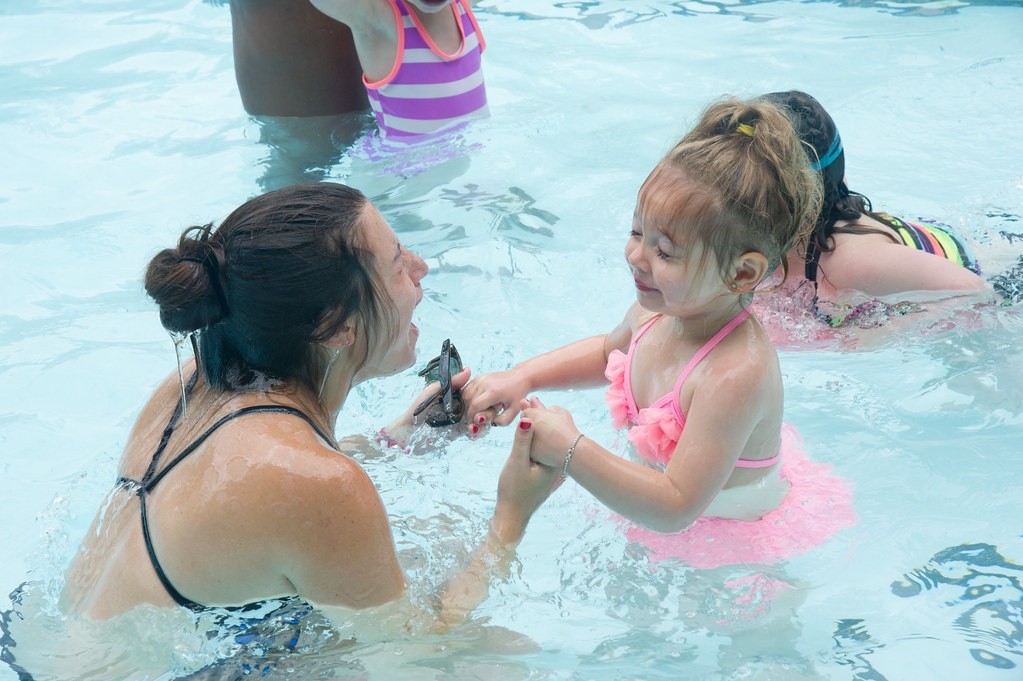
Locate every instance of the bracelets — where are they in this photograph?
[562,434,583,474]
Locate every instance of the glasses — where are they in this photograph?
[413,339,464,427]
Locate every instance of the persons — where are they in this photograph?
[67,181,571,621]
[204,0,371,117]
[311,0,486,135]
[754,89,986,296]
[459,95,822,534]
